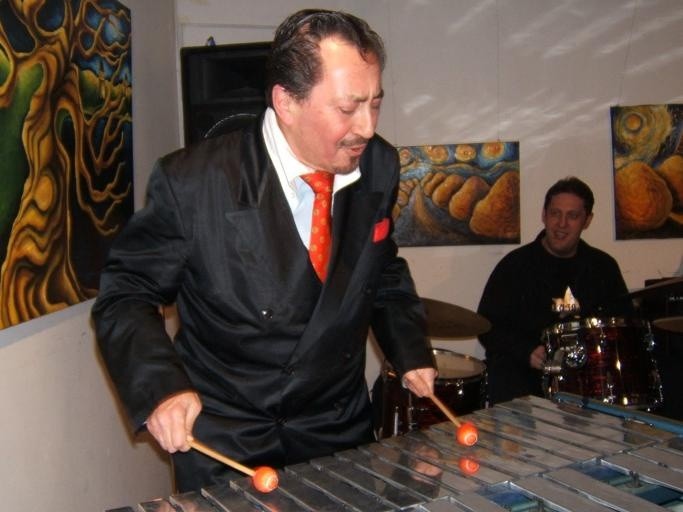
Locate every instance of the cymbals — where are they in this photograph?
[620,277,683,299]
[419,297,492,338]
[653,317,682,332]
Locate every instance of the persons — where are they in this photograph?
[92,5,443,497]
[469,172,635,409]
[142,429,443,512]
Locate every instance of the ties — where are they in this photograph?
[300,168,334,284]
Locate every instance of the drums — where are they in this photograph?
[540,315,664,412]
[372,347,486,442]
